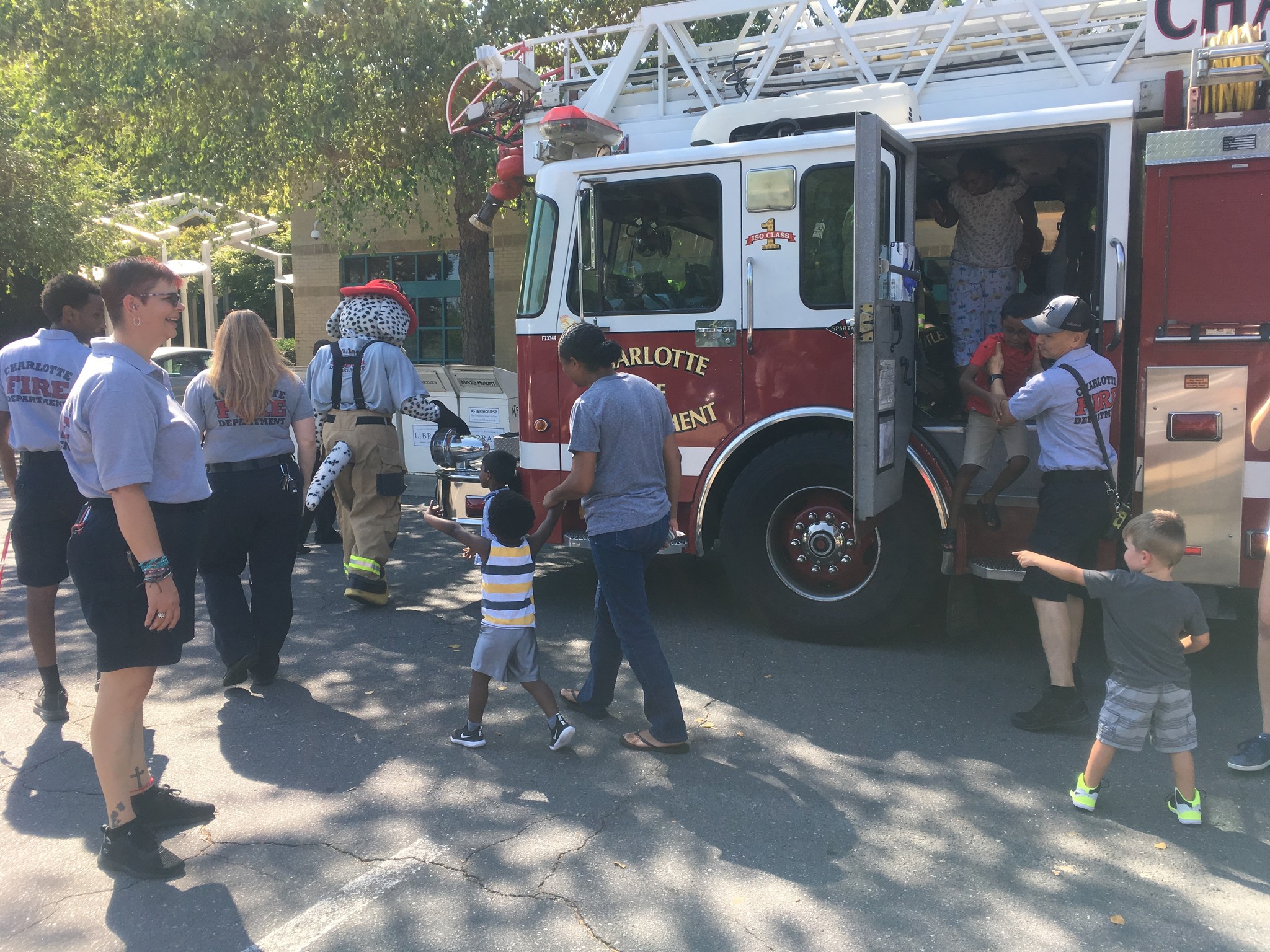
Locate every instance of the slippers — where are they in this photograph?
[938,528,957,552]
[559,687,610,720]
[977,498,1002,530]
[619,733,690,753]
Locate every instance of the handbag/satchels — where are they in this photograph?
[1103,502,1130,544]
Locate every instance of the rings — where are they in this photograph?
[1023,258,1025,259]
[155,611,166,619]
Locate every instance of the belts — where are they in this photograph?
[206,453,293,474]
[324,413,396,427]
[20,451,67,464]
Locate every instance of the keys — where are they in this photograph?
[280,475,293,491]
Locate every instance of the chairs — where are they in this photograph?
[1038,209,1088,304]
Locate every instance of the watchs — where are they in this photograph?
[987,374,1004,387]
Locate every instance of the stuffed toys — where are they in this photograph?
[306,280,470,607]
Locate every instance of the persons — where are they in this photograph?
[917,152,1038,422]
[1022,210,1096,295]
[181,309,317,687]
[543,325,690,755]
[417,451,529,566]
[58,258,215,881]
[987,295,1118,731]
[939,292,1046,552]
[1011,510,1210,824]
[0,274,107,722]
[1226,392,1270,772]
[425,490,575,751]
[295,338,344,554]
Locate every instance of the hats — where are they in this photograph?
[1021,295,1093,334]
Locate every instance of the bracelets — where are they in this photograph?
[136,555,172,592]
[934,214,947,226]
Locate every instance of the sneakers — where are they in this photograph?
[450,725,486,748]
[548,713,575,750]
[97,816,185,879]
[1164,787,1207,824]
[132,783,216,828]
[1227,732,1270,771]
[1069,772,1110,812]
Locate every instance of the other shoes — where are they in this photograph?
[33,685,69,719]
[345,578,389,606]
[1043,669,1084,692]
[249,669,276,685]
[1010,689,1090,732]
[314,530,344,544]
[95,671,101,692]
[297,542,311,554]
[223,652,257,687]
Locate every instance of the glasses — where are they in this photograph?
[120,293,179,307]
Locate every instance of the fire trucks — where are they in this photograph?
[430,0,1268,649]
